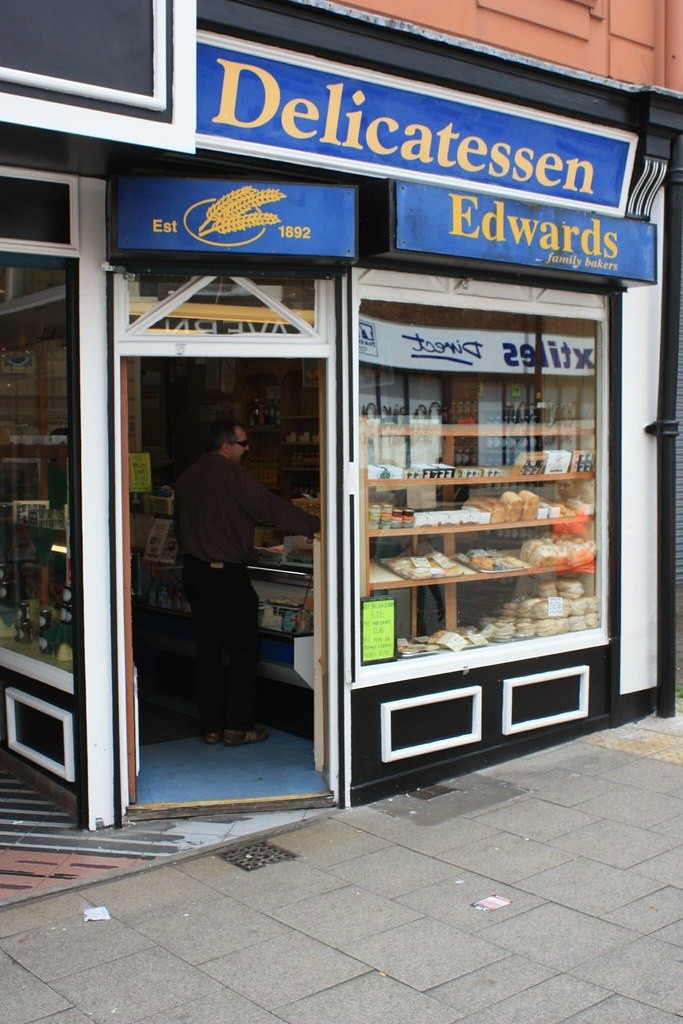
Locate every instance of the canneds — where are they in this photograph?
[15,601,33,643]
[38,608,52,654]
[368,504,415,529]
[60,582,73,624]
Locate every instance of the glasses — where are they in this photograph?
[227,439,249,448]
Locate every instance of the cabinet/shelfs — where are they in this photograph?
[359,413,598,661]
[240,370,320,491]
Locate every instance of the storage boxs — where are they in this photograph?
[367,464,403,480]
[412,512,438,528]
[569,450,596,473]
[567,498,594,515]
[429,463,456,478]
[542,449,572,474]
[449,510,481,525]
[410,464,439,478]
[537,504,548,520]
[429,511,461,526]
[540,502,561,519]
[462,506,491,524]
[508,452,550,474]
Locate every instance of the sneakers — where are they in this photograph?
[204,729,267,746]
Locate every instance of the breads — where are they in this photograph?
[386,490,599,653]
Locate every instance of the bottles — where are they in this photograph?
[289,448,319,467]
[247,456,279,486]
[448,400,478,425]
[251,397,281,427]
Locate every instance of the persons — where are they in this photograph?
[173,419,321,748]
[23,425,72,568]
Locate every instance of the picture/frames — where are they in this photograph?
[302,358,318,388]
